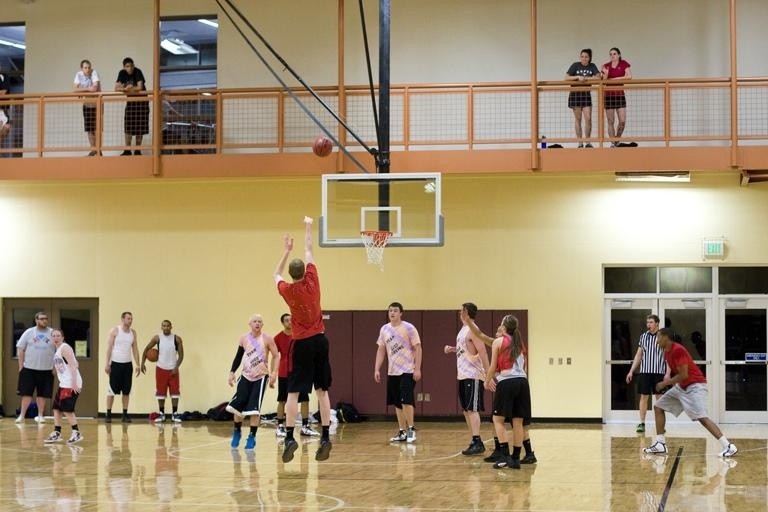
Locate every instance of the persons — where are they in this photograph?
[566,48,600,149]
[0,78,12,145]
[141,320,184,423]
[72,59,103,157]
[462,307,537,464]
[484,313,529,471]
[43,329,85,445]
[161,96,188,156]
[599,47,632,148]
[444,301,497,455]
[273,313,321,437]
[14,312,56,423]
[228,312,280,451]
[625,314,671,434]
[372,301,424,444]
[103,312,140,422]
[642,328,739,457]
[114,55,149,155]
[273,215,334,465]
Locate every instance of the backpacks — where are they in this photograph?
[183,399,369,423]
[15,402,39,419]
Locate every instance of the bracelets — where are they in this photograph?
[136,365,140,369]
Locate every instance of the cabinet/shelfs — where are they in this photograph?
[318,310,528,421]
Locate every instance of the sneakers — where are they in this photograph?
[636,422,646,433]
[718,444,737,457]
[231,425,332,463]
[644,442,667,454]
[14,411,182,445]
[460,442,538,467]
[389,425,417,444]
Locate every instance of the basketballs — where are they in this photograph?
[146,348,158,362]
[312,137,332,157]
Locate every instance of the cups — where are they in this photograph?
[540,135,546,149]
